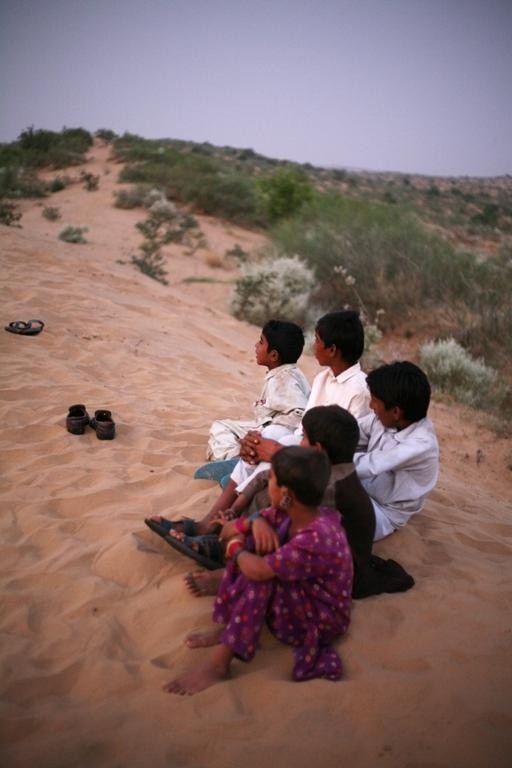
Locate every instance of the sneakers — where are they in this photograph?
[90,410,115,440]
[66,405,89,434]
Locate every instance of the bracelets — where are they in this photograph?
[235,516,248,535]
[227,538,245,565]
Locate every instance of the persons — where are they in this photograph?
[193,310,375,487]
[181,403,376,599]
[159,444,355,697]
[144,360,440,570]
[205,320,311,460]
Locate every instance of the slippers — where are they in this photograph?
[5,318,45,334]
[163,533,225,571]
[145,514,198,537]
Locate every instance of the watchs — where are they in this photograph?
[248,510,261,528]
